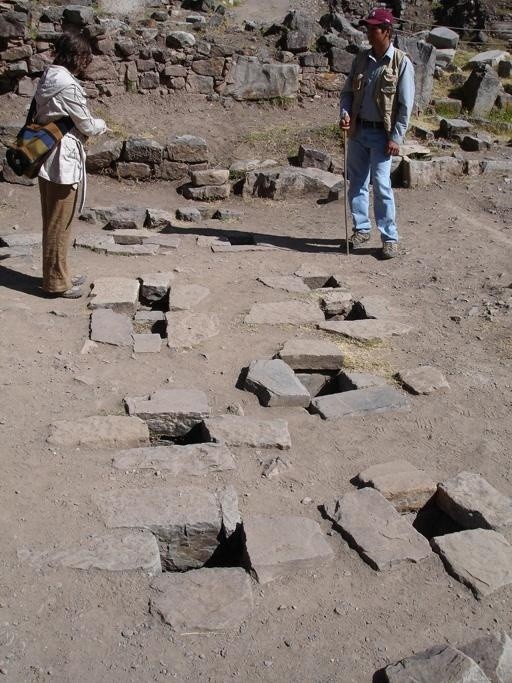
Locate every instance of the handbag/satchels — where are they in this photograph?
[5,118,76,179]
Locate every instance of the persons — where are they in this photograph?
[339,8,416,259]
[32,30,113,298]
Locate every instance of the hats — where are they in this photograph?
[358,9,394,29]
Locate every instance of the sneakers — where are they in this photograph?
[381,241,398,258]
[348,231,370,247]
[57,271,86,299]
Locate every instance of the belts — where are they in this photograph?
[356,117,385,129]
